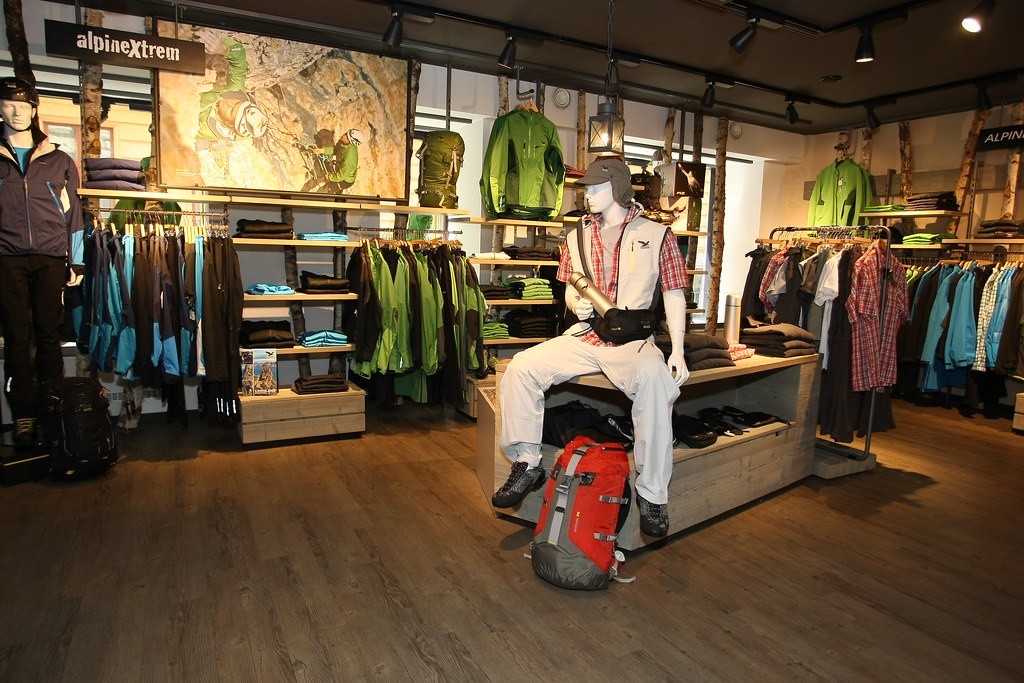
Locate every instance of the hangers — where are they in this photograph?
[519,88,540,112]
[356,227,464,254]
[897,253,1024,276]
[754,226,885,251]
[92,208,229,243]
[834,143,849,161]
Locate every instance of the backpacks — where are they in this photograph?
[530,435,636,591]
[59,383,118,476]
[416,130,465,210]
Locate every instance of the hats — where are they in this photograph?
[575,160,635,204]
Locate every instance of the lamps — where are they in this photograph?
[854,21,876,63]
[962,0,995,32]
[497,34,519,69]
[380,8,404,46]
[700,76,716,109]
[786,97,798,124]
[728,7,761,54]
[587,0,624,156]
[865,102,881,130]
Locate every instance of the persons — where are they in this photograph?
[0,77,84,446]
[491,159,690,539]
[313,130,363,193]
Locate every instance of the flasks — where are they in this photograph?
[568,273,618,319]
[722,294,741,343]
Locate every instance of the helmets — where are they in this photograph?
[0,77,40,108]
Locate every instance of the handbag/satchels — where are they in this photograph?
[585,308,657,345]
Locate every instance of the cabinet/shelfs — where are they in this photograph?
[445,176,710,418]
[474,348,826,551]
[220,239,366,443]
[857,211,1024,254]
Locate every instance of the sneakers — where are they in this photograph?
[11,418,38,445]
[635,495,668,538]
[492,462,547,508]
